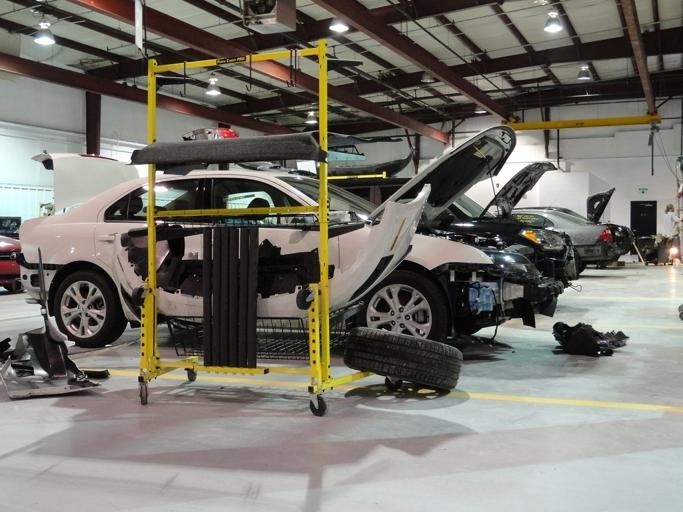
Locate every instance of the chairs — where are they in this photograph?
[241,198,271,221]
[119,196,143,219]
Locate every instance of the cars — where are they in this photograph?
[15,124,565,348]
[236,160,577,320]
[491,187,635,275]
[0,235,21,291]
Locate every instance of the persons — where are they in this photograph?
[661,204,683,265]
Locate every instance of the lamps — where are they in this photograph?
[542,6,592,81]
[304,109,317,125]
[327,19,350,34]
[203,73,220,97]
[33,7,55,47]
